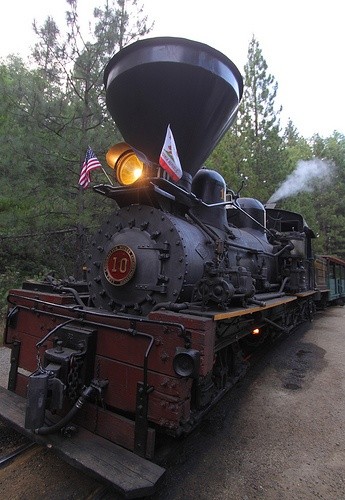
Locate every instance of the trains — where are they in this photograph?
[0,36,345,500]
